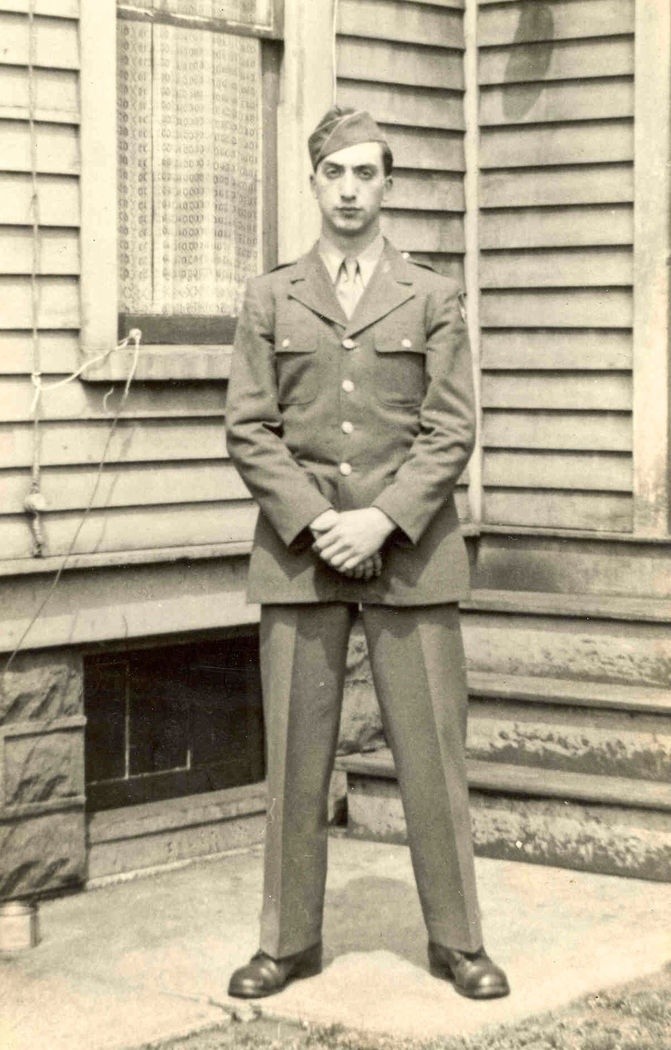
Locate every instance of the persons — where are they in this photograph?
[223,105,510,999]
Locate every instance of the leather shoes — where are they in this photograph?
[230,943,321,998]
[428,943,510,998]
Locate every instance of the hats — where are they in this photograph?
[308,107,386,172]
